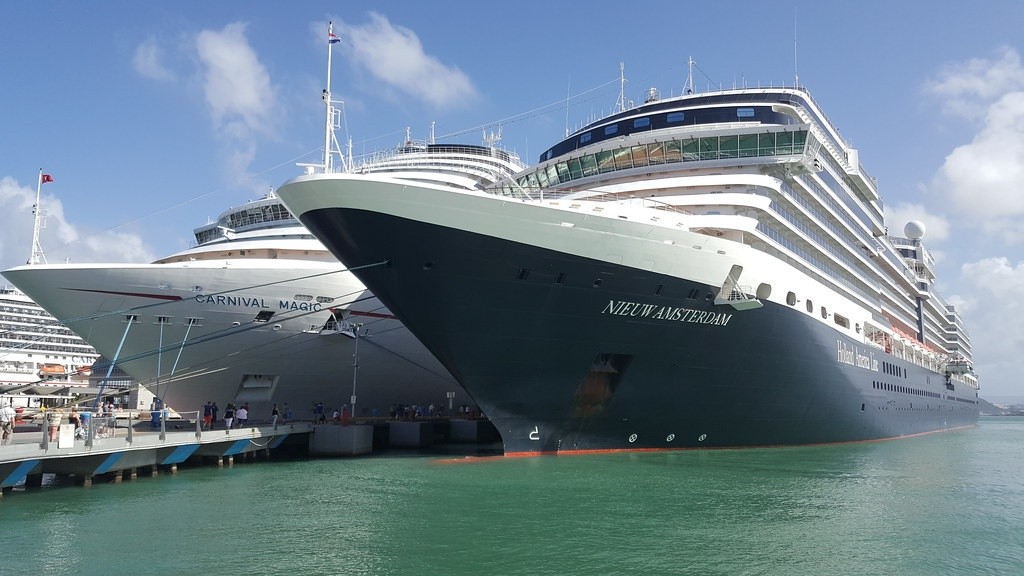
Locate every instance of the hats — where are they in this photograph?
[4,402,11,406]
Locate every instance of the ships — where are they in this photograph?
[276,7,980,456]
[0,22,530,425]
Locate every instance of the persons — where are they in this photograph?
[272,403,280,430]
[333,405,347,424]
[49,408,64,442]
[95,401,115,417]
[222,402,249,430]
[202,401,219,430]
[391,402,445,422]
[69,407,81,438]
[281,403,289,425]
[312,402,328,424]
[459,404,486,420]
[0,402,15,444]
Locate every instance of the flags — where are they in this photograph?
[329,33,341,44]
[42,175,54,184]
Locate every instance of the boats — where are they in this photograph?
[0,167,181,408]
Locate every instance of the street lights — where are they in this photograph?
[350,323,363,417]
